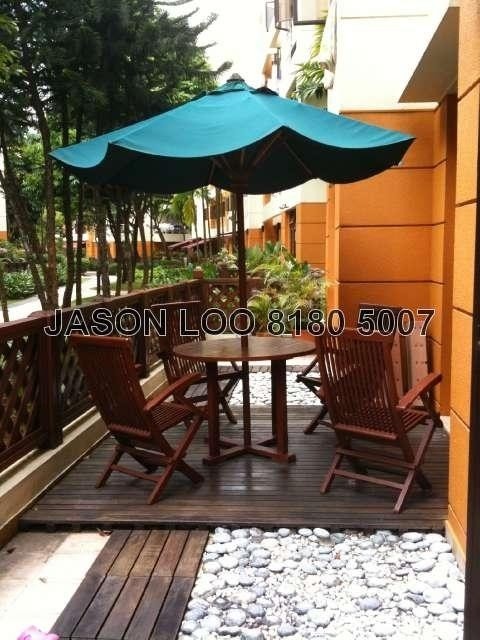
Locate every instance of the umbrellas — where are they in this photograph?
[44,71,417,447]
